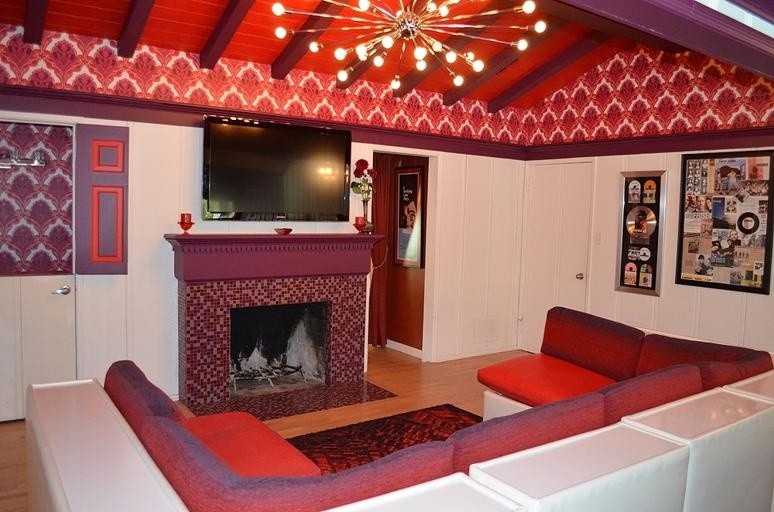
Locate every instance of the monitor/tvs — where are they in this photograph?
[202,112,353,224]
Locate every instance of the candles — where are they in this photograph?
[181,214,191,223]
[355,217,364,225]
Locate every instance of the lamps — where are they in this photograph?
[270,0,547,91]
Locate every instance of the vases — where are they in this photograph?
[360,199,373,236]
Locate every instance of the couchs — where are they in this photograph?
[26,304,774,511]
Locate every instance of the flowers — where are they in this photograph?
[350,158,379,202]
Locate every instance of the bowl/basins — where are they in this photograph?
[274,227,295,236]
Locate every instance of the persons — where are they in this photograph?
[684,157,769,278]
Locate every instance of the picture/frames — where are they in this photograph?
[674,148,773,296]
[613,170,667,299]
[391,165,426,271]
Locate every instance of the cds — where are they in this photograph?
[624,178,656,288]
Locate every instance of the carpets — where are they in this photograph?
[287,402,483,478]
